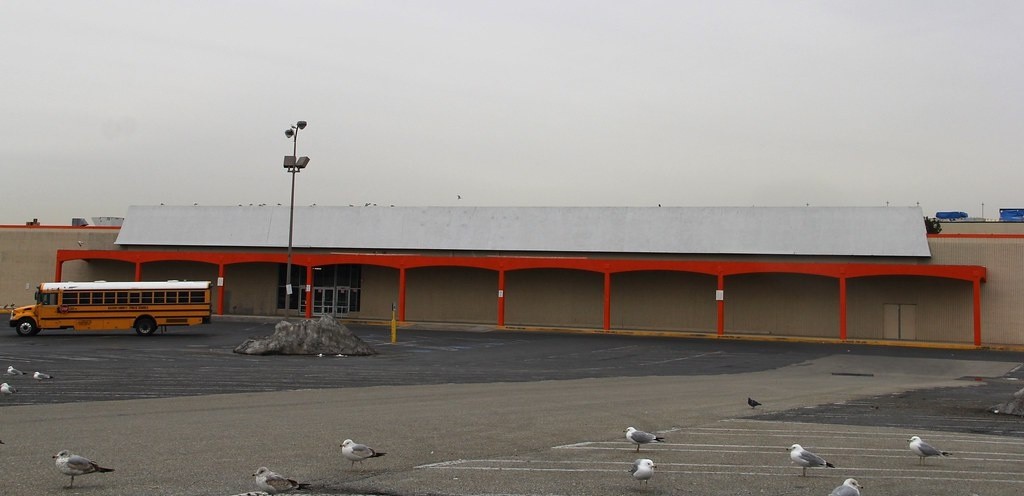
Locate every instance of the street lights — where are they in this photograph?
[285,121,309,320]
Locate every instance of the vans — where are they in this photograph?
[9,280,212,337]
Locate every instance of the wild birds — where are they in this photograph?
[906,435,945,465]
[628,458,657,486]
[786,443,835,477]
[0,383,16,394]
[6,365,24,376]
[747,397,762,408]
[252,467,312,494]
[51,449,116,486]
[339,439,388,467]
[623,427,666,452]
[33,371,53,381]
[827,478,863,496]
